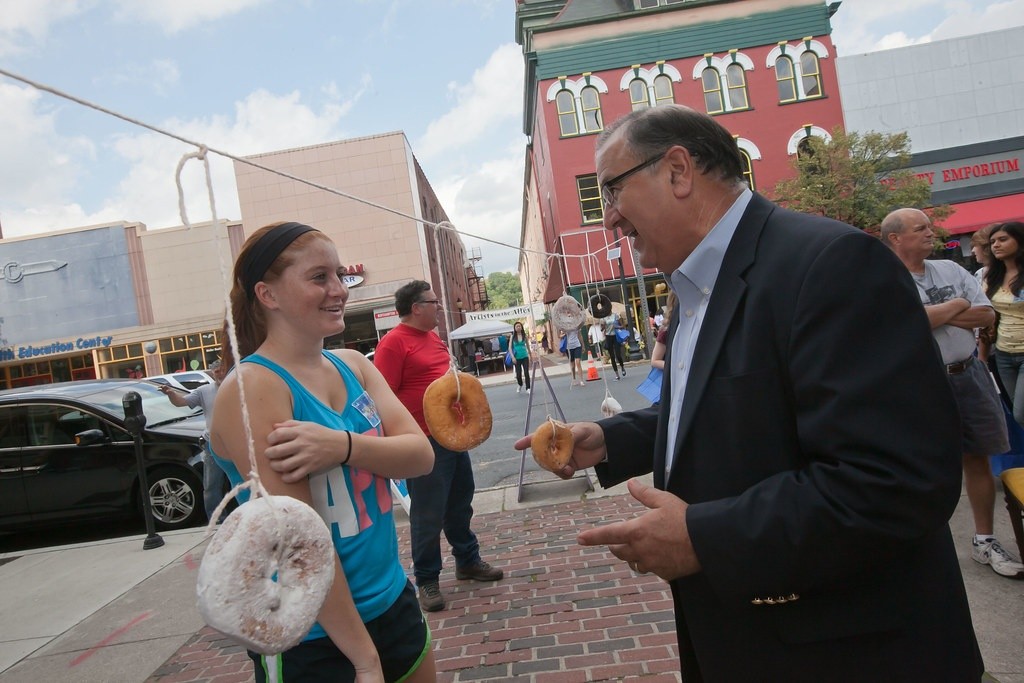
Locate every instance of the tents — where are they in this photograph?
[449,320,514,340]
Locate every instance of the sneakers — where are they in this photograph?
[456,560,503,581]
[971,534,1024,578]
[419,581,446,612]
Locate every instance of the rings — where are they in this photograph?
[635,562,639,572]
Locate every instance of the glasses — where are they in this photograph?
[214,369,224,373]
[601,153,664,210]
[418,300,440,304]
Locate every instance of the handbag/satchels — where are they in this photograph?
[559,336,567,352]
[504,334,517,367]
[614,313,630,343]
[604,344,609,350]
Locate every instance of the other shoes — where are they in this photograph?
[580,382,585,386]
[517,385,521,392]
[573,379,576,385]
[526,389,531,394]
[623,370,626,376]
[613,377,621,381]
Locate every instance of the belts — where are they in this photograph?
[944,351,979,374]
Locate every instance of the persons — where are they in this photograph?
[208,221,437,683]
[969,222,1024,427]
[513,104,984,683]
[588,324,607,361]
[541,333,548,352]
[560,329,586,386]
[649,309,664,328]
[373,280,503,612]
[879,207,1024,578]
[650,290,678,370]
[158,359,227,525]
[509,322,532,394]
[466,338,476,365]
[600,310,627,379]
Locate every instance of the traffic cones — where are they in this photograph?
[585,350,602,381]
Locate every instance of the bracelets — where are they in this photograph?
[340,430,352,464]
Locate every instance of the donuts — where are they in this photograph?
[551,296,587,331]
[423,372,494,452]
[197,495,336,656]
[531,421,574,471]
[600,397,622,418]
[587,294,613,318]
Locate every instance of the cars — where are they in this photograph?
[139,369,219,394]
[0,378,207,548]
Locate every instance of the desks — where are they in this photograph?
[475,356,506,376]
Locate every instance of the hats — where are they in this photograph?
[208,359,222,369]
